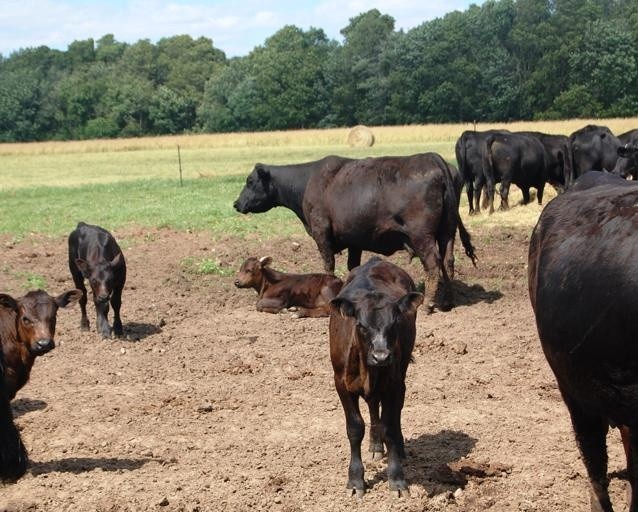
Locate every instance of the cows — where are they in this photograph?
[232,154,481,316]
[454,124,638,216]
[67,220,127,342]
[526,170,638,512]
[327,254,425,498]
[233,254,344,318]
[0,287,83,485]
